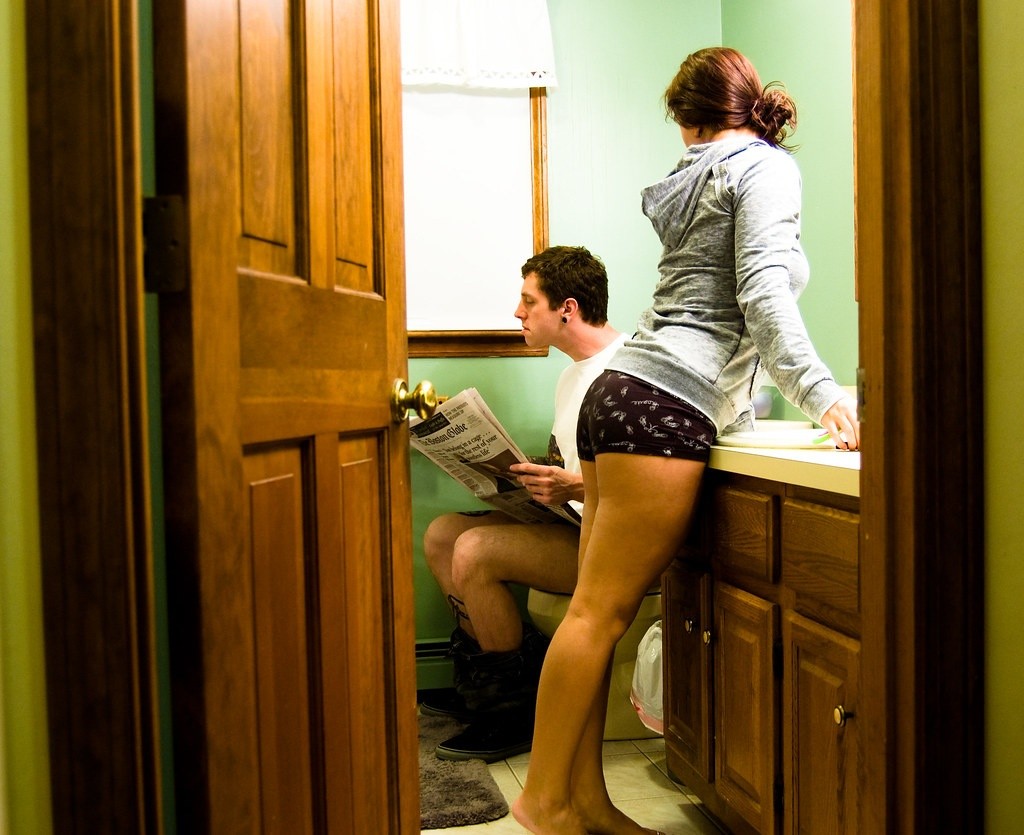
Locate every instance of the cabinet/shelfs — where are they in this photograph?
[660,420,862,835]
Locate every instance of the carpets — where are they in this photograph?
[415,696,513,830]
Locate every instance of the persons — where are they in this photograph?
[512,47,861,834]
[422,244,634,765]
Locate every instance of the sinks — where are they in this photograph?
[715,428,847,450]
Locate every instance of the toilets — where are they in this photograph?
[527,586,671,742]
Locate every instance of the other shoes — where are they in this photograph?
[420,690,465,713]
[435,725,533,764]
